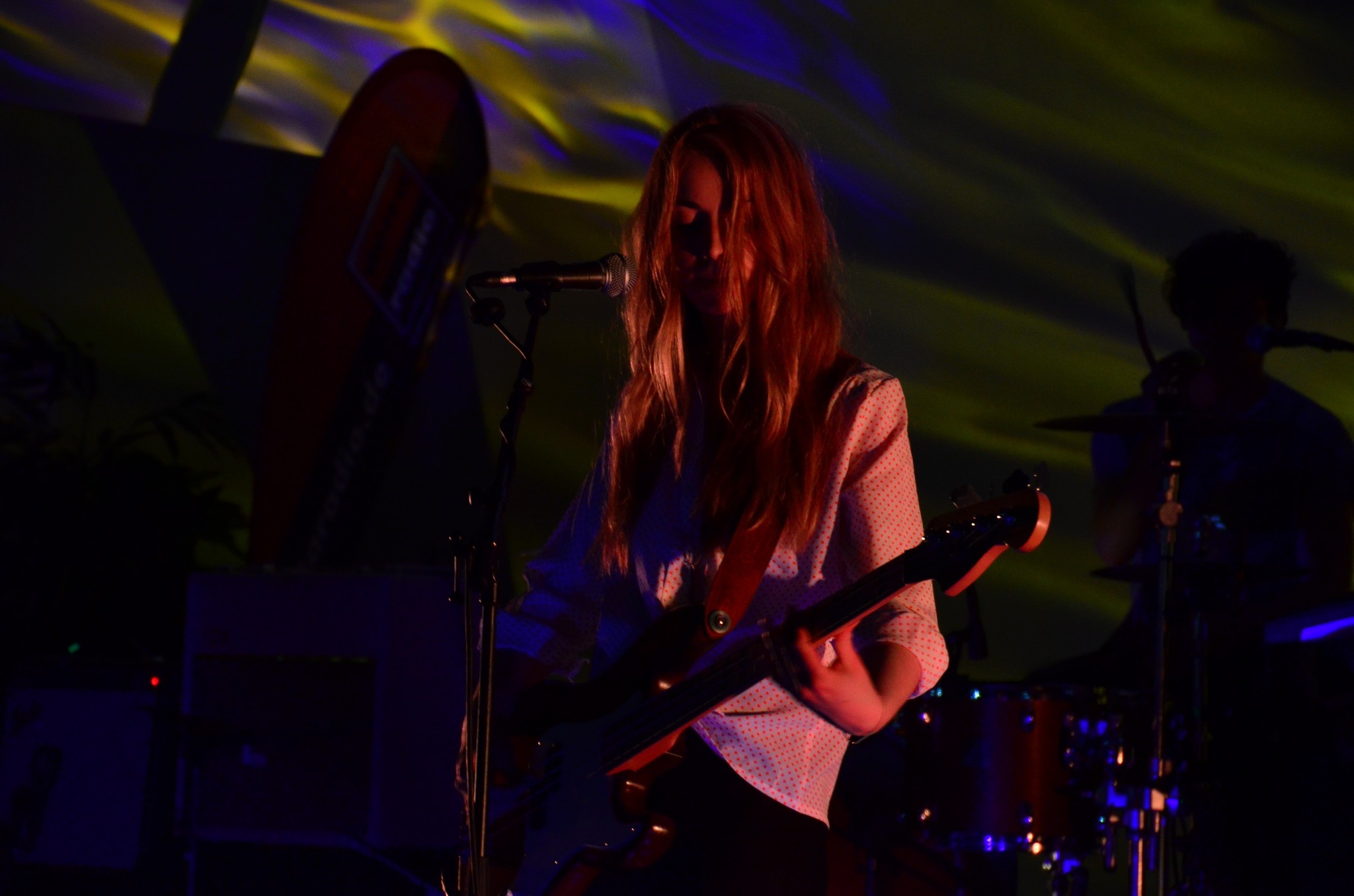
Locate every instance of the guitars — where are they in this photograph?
[440,467,1052,896]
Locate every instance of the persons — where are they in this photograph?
[461,100,951,896]
[1091,223,1353,896]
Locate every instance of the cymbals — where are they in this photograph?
[1094,564,1161,587]
[1034,413,1299,433]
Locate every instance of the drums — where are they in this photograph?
[883,682,1076,877]
[1056,710,1182,842]
